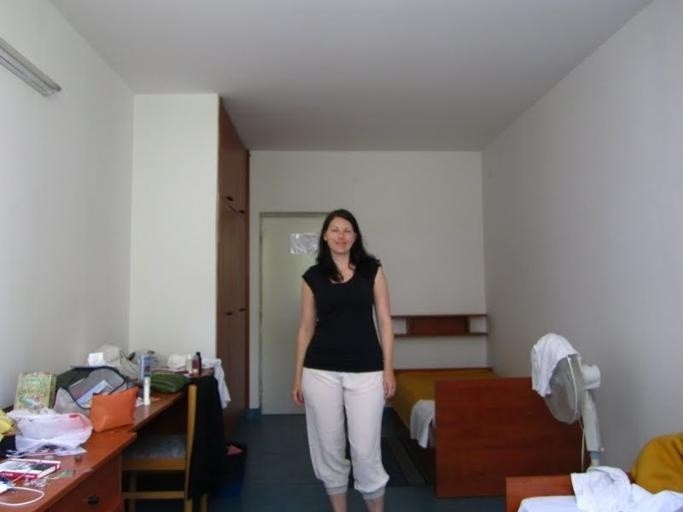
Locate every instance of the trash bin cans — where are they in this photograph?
[211,440,248,497]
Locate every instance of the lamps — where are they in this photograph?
[0,38,62,98]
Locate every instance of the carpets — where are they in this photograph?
[346,438,428,487]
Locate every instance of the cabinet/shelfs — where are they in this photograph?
[218,98,249,442]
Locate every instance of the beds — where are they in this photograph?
[384,313,593,499]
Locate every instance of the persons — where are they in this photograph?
[290,208,397,512]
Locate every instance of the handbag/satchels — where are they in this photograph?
[149,370,189,392]
[198,440,246,498]
[90,385,139,432]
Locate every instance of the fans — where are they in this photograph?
[530,332,603,470]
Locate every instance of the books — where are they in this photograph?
[12,371,57,409]
[0,458,61,480]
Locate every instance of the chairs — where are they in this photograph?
[506,429,683,512]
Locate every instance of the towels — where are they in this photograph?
[530,332,583,398]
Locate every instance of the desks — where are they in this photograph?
[0,364,215,512]
